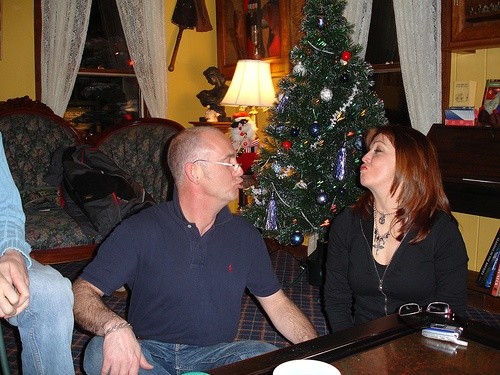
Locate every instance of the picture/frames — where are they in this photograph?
[216,0,289,78]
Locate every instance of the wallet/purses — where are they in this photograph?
[403,305,500,350]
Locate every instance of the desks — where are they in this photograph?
[201,313,500,375]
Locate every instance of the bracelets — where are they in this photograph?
[104,323,133,335]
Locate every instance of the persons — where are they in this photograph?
[72,126,320,375]
[0,131,74,375]
[196,66,230,118]
[321,125,469,333]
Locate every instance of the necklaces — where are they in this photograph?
[372,201,404,255]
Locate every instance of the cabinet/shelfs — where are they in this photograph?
[441,0,500,54]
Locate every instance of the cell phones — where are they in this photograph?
[423,338,467,354]
[421,323,469,346]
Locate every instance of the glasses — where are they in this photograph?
[399,301,451,318]
[193,159,243,175]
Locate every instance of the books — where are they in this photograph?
[478,226,500,296]
[450,80,478,107]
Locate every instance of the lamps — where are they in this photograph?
[220,59,276,125]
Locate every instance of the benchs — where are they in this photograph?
[0,95,186,265]
